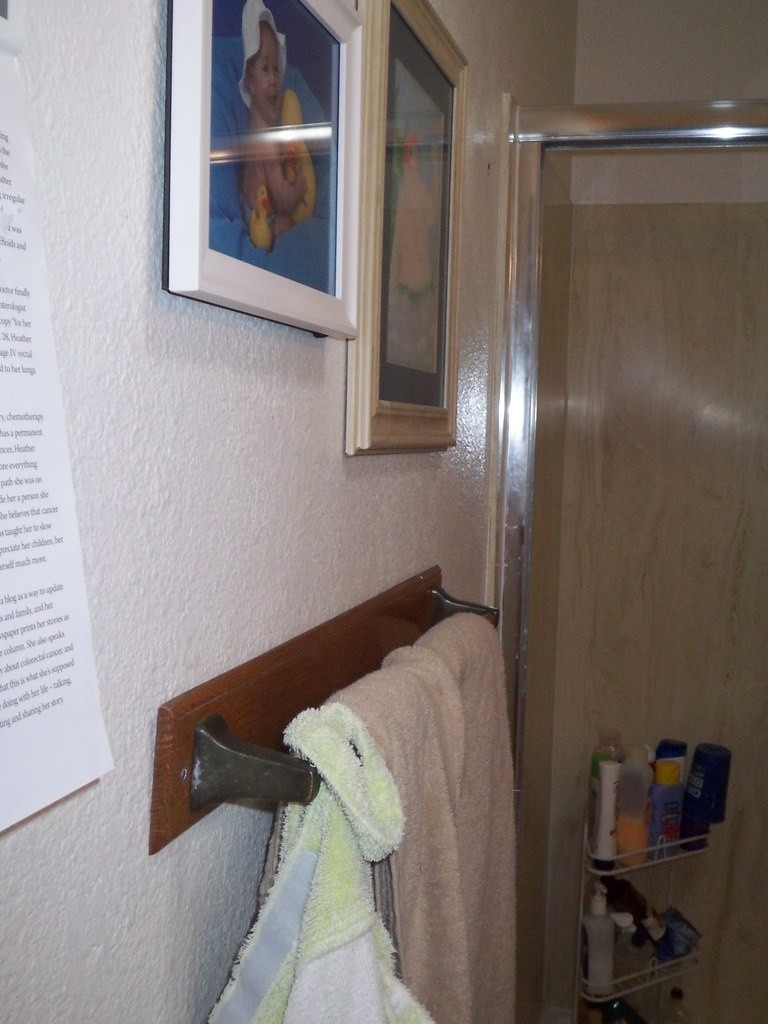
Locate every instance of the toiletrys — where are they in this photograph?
[579,720,735,1005]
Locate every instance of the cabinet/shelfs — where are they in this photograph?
[572,805,714,1024]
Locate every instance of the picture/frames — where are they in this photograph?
[161,0,371,341]
[343,0,470,458]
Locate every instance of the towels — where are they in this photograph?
[206,700,434,1024]
[323,606,523,1024]
